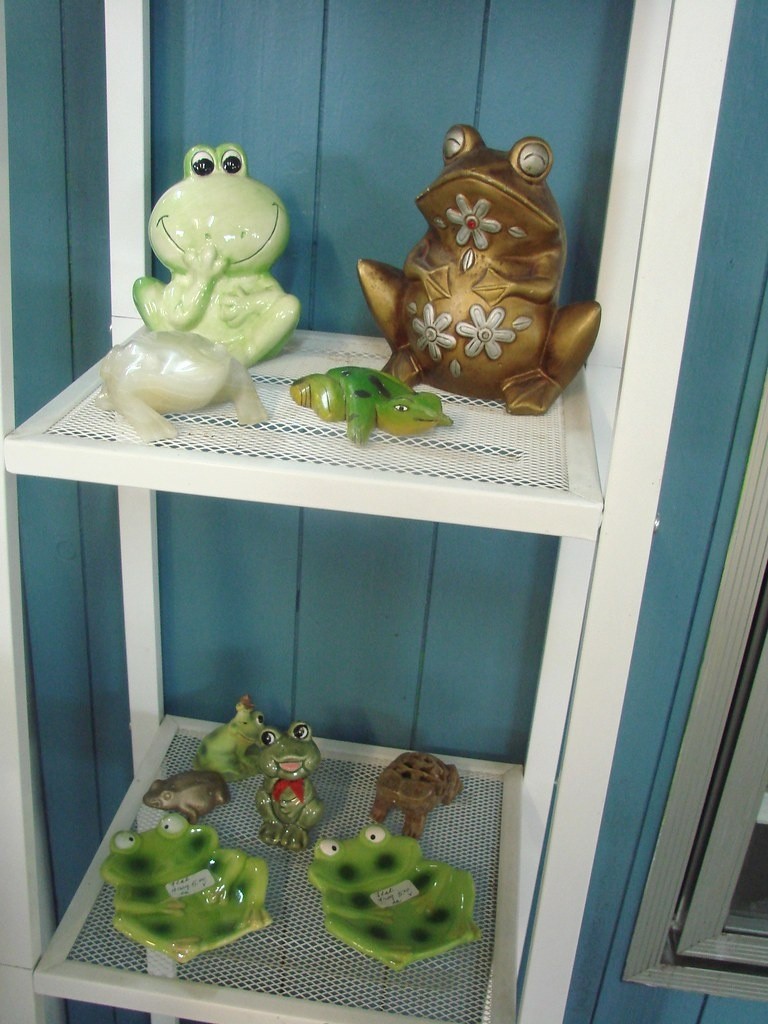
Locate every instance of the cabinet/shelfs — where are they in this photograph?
[0,0,735,1024]
